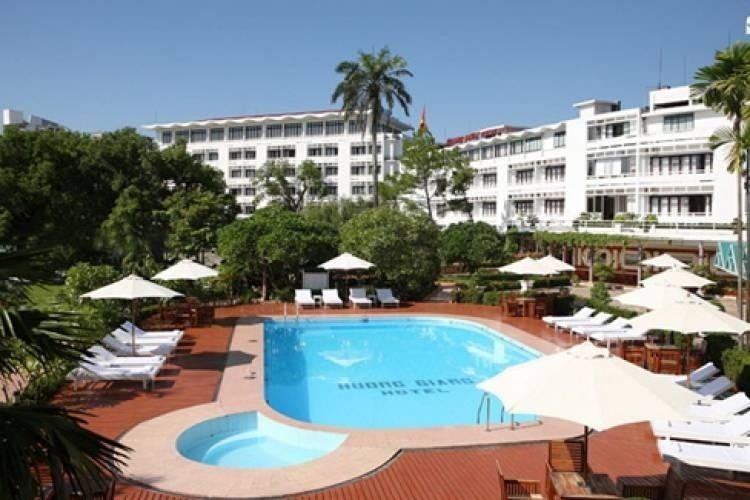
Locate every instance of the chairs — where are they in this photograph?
[652,361,750,474]
[500,291,554,320]
[541,304,642,359]
[619,336,697,375]
[375,288,401,310]
[65,321,186,395]
[615,465,688,500]
[348,287,373,310]
[321,288,345,310]
[494,459,544,500]
[294,289,316,312]
[544,439,592,500]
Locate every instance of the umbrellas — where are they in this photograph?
[149,260,222,305]
[79,274,185,357]
[612,250,750,392]
[317,251,377,302]
[497,253,576,301]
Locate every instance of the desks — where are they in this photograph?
[313,295,322,308]
[546,471,624,500]
